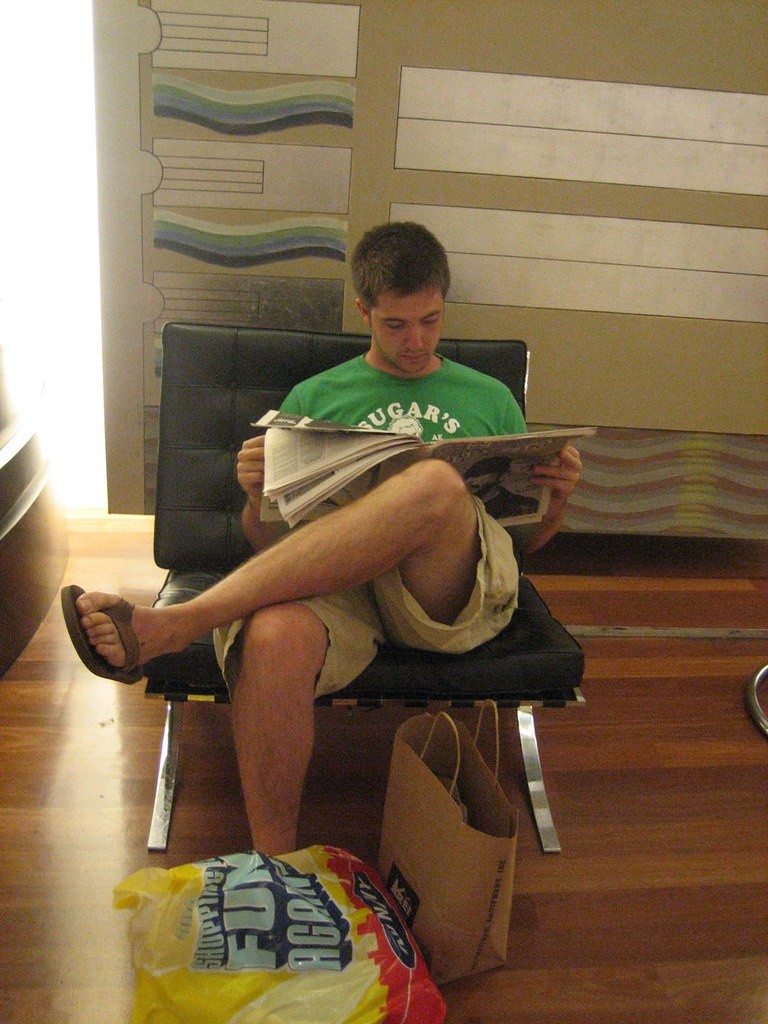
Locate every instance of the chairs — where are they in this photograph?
[144,321,587,854]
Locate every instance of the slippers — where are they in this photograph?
[61,584,145,686]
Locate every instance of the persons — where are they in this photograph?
[62,220,583,859]
[464,457,539,520]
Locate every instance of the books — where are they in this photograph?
[250,410,597,527]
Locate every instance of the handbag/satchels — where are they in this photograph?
[378,700,519,988]
